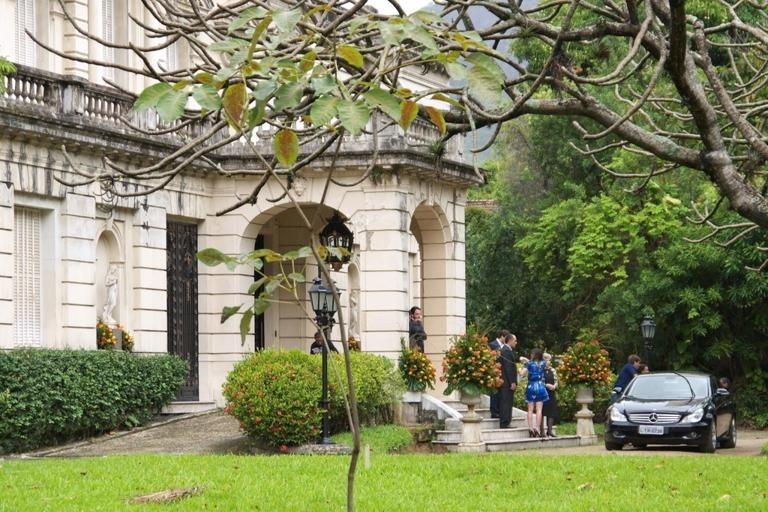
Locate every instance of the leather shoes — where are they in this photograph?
[500,423,516,428]
[491,413,501,417]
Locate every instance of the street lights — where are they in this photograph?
[639,309,658,372]
[308,277,340,445]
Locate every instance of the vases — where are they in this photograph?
[458,386,482,421]
[575,380,594,414]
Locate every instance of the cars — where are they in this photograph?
[600,371,746,453]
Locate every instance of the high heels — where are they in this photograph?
[529,429,541,437]
[540,431,558,437]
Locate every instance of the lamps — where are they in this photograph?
[318,209,355,273]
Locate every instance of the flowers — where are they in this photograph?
[96,321,135,352]
[396,338,438,394]
[438,321,505,399]
[553,326,613,396]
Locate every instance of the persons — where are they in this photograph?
[489,329,559,437]
[409,306,427,353]
[638,363,650,374]
[717,378,731,395]
[310,333,338,355]
[610,355,641,406]
